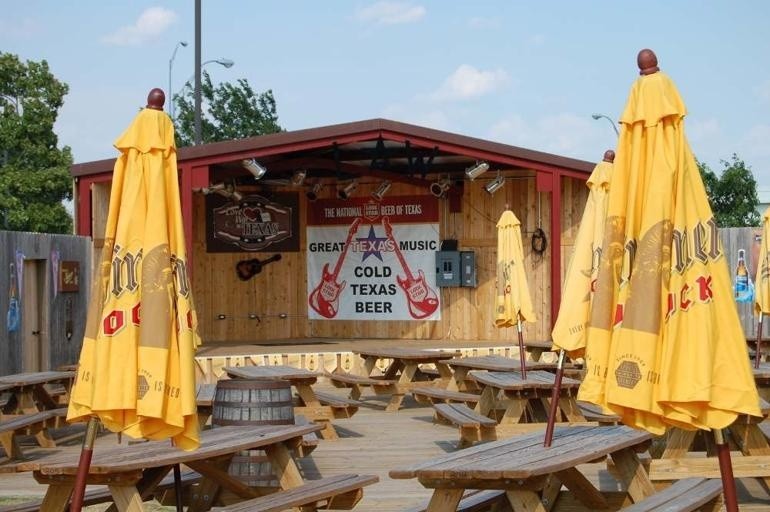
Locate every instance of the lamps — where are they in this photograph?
[289,168,308,186]
[193,180,246,205]
[429,172,453,197]
[305,180,394,203]
[240,157,268,181]
[465,159,507,196]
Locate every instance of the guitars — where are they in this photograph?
[383,215,438,318]
[237,255,281,280]
[309,218,362,318]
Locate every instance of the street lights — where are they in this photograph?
[169,40,234,118]
[592,113,619,138]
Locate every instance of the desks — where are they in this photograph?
[0,425,328,477]
[388,425,656,512]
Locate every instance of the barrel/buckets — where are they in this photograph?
[212,379,294,487]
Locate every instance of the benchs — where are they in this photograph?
[1,364,77,458]
[392,489,505,512]
[194,364,361,438]
[666,340,770,457]
[332,342,619,443]
[615,477,725,512]
[0,471,201,512]
[208,473,379,512]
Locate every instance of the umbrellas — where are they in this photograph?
[753,206,770,369]
[576,48,762,512]
[66,88,202,512]
[544,151,615,447]
[494,204,537,380]
